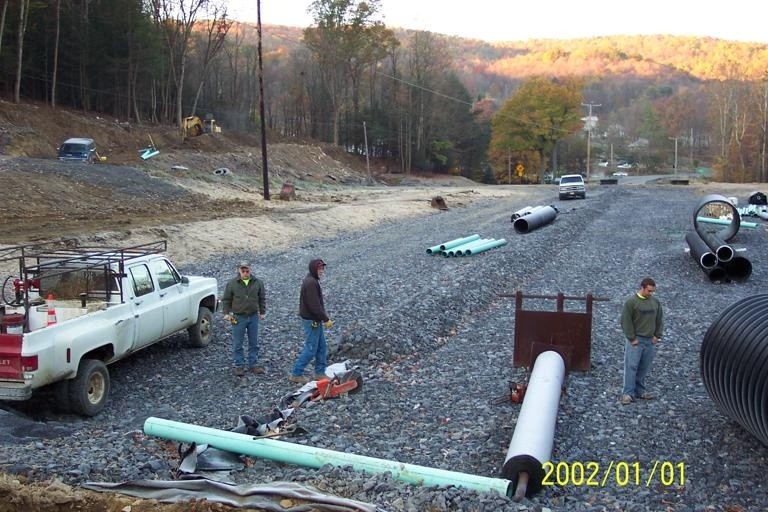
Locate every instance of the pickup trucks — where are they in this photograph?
[0,238,219,418]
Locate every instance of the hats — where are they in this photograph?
[316,259,326,268]
[240,263,250,269]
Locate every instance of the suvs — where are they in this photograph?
[558,174,586,200]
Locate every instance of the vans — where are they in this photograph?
[56,137,98,165]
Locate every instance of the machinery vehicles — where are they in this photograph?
[177,117,223,138]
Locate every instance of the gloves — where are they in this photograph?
[324,320,334,329]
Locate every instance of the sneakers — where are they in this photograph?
[637,391,654,400]
[250,367,263,374]
[313,372,327,381]
[621,393,632,405]
[234,367,245,376]
[288,374,308,383]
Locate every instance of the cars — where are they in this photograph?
[611,171,628,177]
[618,163,632,169]
[544,175,553,183]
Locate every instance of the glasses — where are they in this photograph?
[241,270,249,272]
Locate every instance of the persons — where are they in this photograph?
[289,259,333,382]
[222,263,266,376]
[620,278,664,404]
